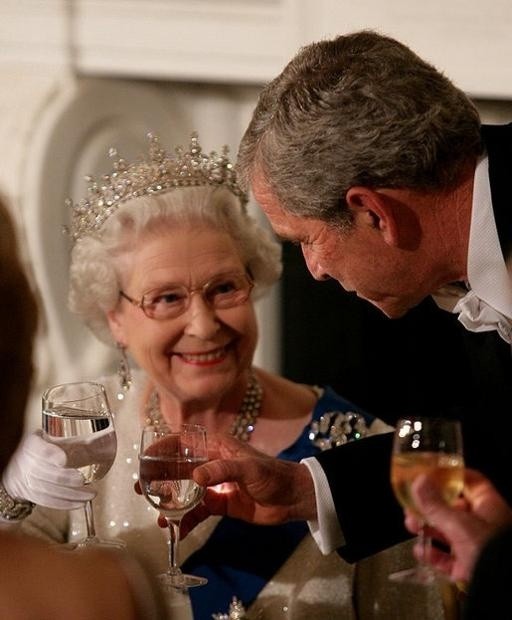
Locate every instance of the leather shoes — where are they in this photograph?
[1,428,97,511]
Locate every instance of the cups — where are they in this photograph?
[120,270,255,321]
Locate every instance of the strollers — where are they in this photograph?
[452,291,511,345]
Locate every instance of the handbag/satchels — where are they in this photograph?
[0,481,37,523]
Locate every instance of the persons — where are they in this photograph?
[401,459,511,619]
[0,127,457,619]
[130,28,511,568]
[0,188,160,619]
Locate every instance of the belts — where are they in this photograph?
[391,415,467,587]
[40,382,125,552]
[135,424,209,589]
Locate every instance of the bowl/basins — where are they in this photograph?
[143,368,267,446]
